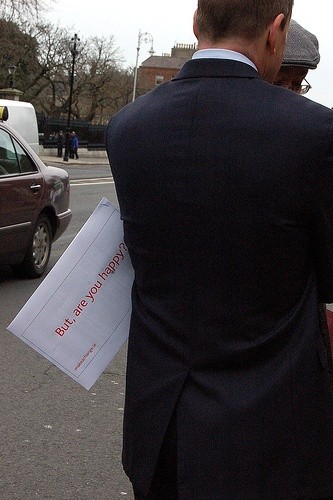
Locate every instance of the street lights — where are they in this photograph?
[64,32,81,161]
[132,28,155,102]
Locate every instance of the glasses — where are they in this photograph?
[271,78,311,94]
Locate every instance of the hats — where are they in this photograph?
[279,19,320,70]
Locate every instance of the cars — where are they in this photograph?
[0,106,73,280]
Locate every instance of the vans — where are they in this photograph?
[0,101,41,157]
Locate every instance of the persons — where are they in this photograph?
[275,16,320,93]
[105,0,333,500]
[56,131,79,159]
